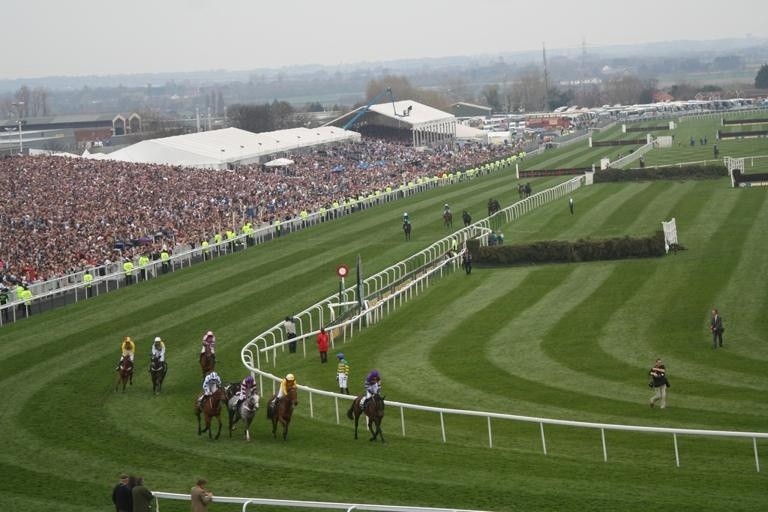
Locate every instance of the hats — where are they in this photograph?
[119,473,128,479]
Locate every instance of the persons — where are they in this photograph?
[649,358,668,410]
[236,374,256,410]
[713,143,720,158]
[270,373,296,418]
[710,307,724,351]
[132,478,155,512]
[112,473,133,510]
[116,336,136,371]
[1,116,602,319]
[190,478,213,512]
[148,337,167,374]
[689,135,709,147]
[360,369,381,410]
[198,331,220,365]
[638,154,645,168]
[335,353,350,395]
[197,371,222,408]
[316,328,329,363]
[284,315,298,354]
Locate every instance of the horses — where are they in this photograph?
[346,394,388,444]
[199,343,215,381]
[193,383,229,441]
[267,385,299,441]
[225,390,261,442]
[444,209,452,229]
[463,211,471,226]
[149,354,168,395]
[115,354,136,394]
[403,221,411,242]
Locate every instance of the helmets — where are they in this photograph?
[245,376,254,384]
[207,331,213,336]
[124,336,130,343]
[154,337,161,342]
[336,353,345,358]
[210,372,218,379]
[372,369,378,376]
[286,373,295,382]
